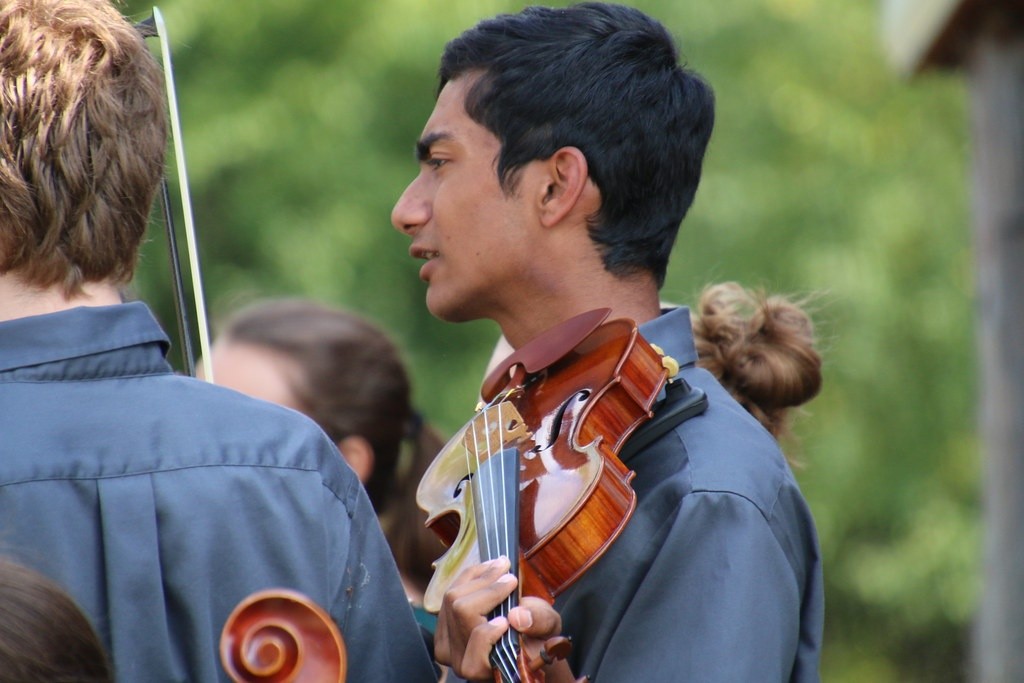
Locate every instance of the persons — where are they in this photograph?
[390,6,822,682]
[1,560,107,683]
[479,277,822,444]
[0,1,439,683]
[190,303,464,680]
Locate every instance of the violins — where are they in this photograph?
[416,308,671,683]
